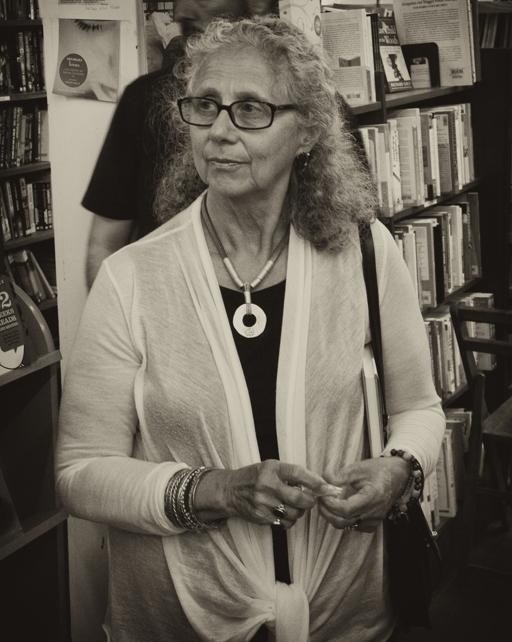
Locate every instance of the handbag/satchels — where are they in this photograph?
[380,503,439,627]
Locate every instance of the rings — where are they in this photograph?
[272,517,282,528]
[273,503,288,518]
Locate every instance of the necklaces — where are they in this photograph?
[200,190,288,339]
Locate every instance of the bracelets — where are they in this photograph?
[165,465,228,535]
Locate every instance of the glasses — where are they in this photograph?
[175,97,305,129]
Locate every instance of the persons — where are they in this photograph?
[58,19,120,103]
[80,0,373,294]
[54,14,446,642]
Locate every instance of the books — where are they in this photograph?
[319,0,497,537]
[0,0,53,377]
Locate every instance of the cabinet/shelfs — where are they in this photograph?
[2,1,76,641]
[37,0,512,642]
